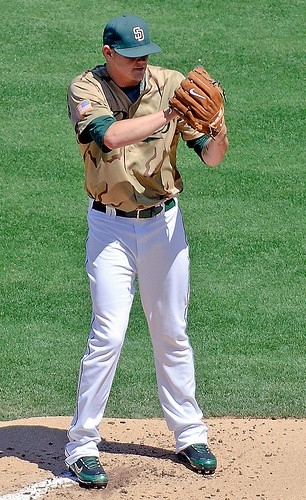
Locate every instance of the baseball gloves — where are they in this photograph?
[168,64,227,140]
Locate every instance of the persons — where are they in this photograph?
[64,13,230,488]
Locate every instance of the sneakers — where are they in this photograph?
[177,443,217,473]
[69,456,108,487]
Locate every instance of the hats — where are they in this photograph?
[103,15,162,57]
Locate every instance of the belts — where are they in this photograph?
[90,200,176,218]
